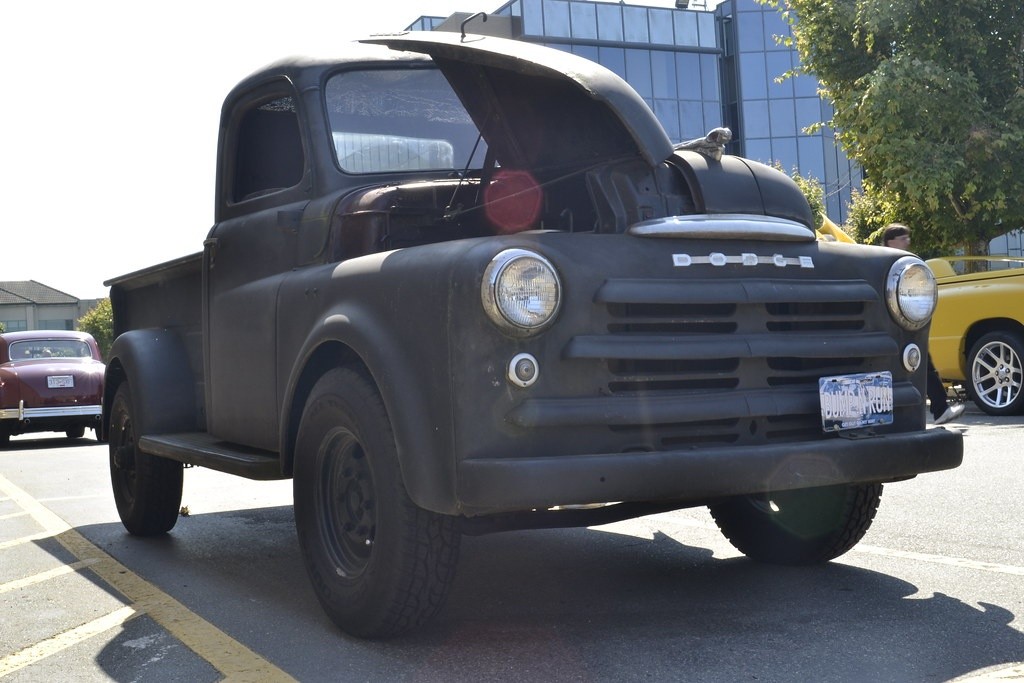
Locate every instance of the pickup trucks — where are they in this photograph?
[100,11,964,646]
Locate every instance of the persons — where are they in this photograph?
[883,223,966,423]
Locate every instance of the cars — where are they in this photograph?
[0,329,106,450]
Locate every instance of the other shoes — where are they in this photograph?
[934,404,965,425]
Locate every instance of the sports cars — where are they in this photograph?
[815,211,1024,414]
